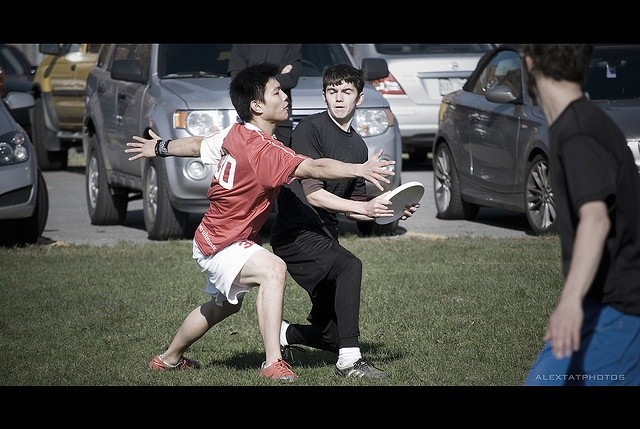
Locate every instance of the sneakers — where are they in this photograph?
[148,355,200,373]
[334,358,390,382]
[259,358,300,383]
[280,319,307,366]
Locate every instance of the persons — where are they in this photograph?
[124,61,397,382]
[518,43,640,387]
[268,62,422,380]
[226,44,302,148]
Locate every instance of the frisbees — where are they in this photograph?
[374,181,424,224]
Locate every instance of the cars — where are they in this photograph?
[433,42,638,235]
[343,42,500,159]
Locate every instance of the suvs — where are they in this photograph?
[1,99,48,242]
[34,42,107,171]
[85,43,403,238]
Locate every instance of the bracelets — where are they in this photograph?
[154,139,173,157]
